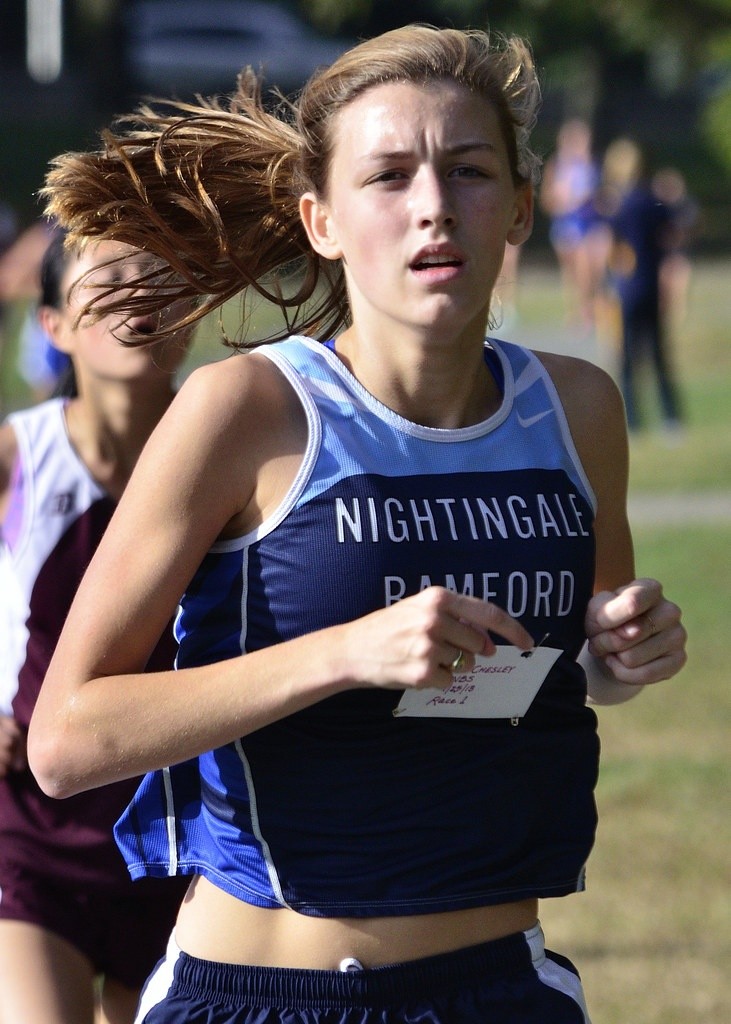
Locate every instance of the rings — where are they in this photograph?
[449,649,466,675]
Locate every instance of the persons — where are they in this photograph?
[0,213,74,399]
[493,114,689,434]
[28,20,689,1024]
[0,219,187,1024]
